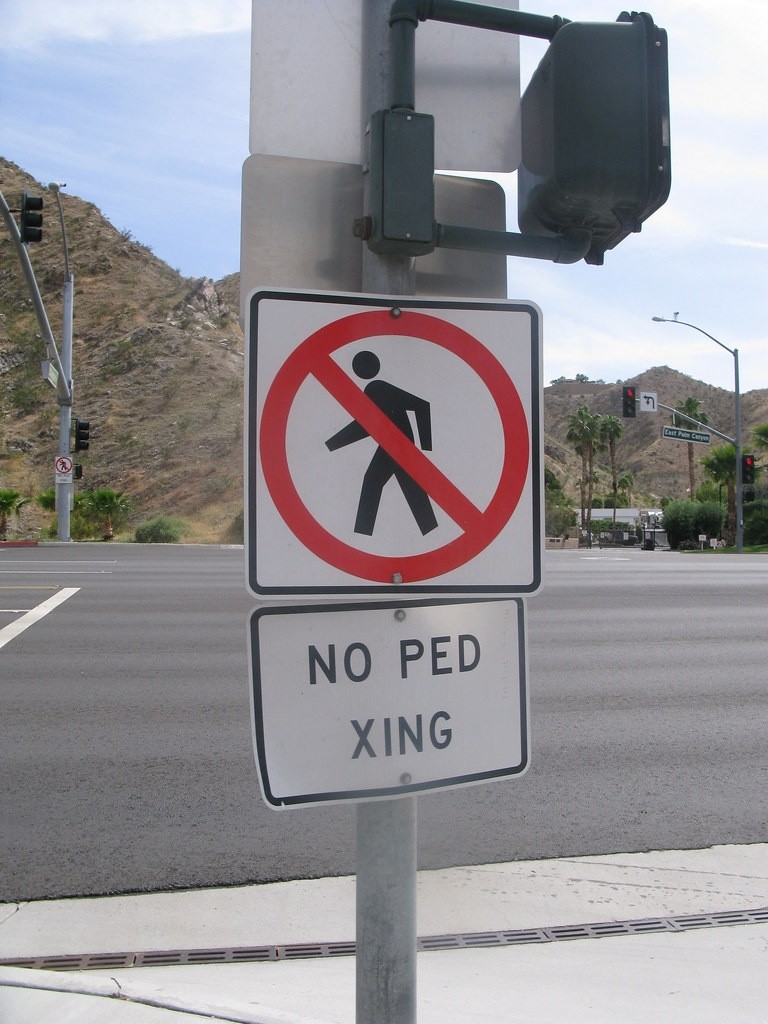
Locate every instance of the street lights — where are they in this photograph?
[48,180,73,543]
[652,317,742,552]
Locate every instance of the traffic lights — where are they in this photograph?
[741,454,755,484]
[74,418,89,451]
[20,194,43,243]
[622,387,636,418]
